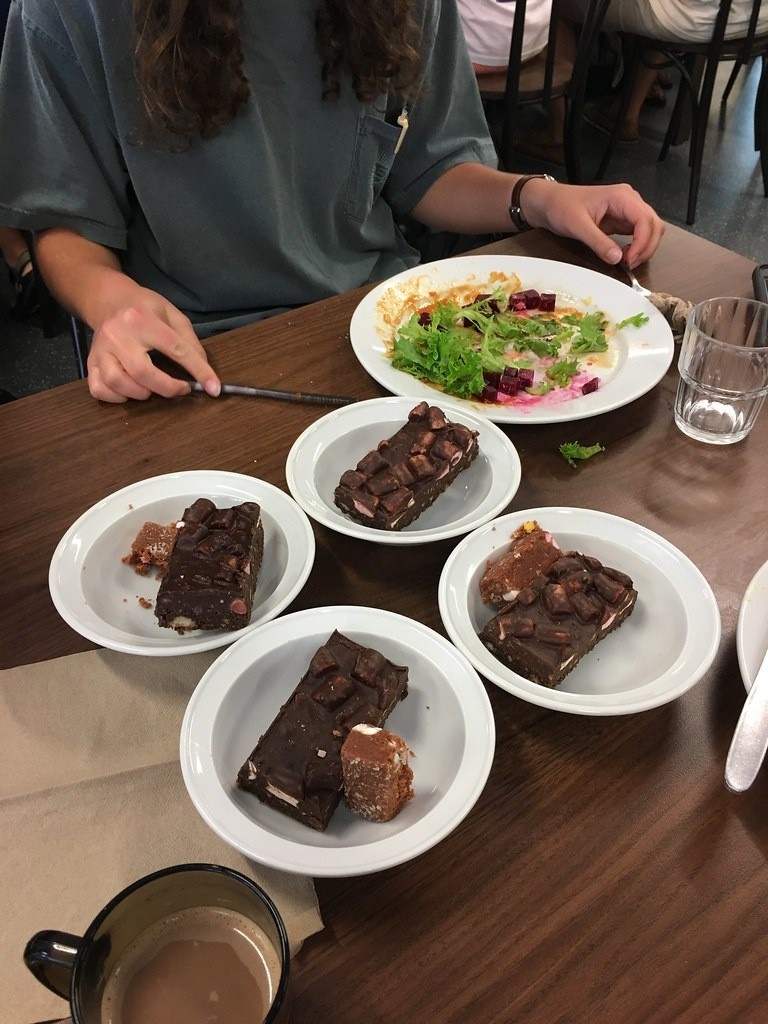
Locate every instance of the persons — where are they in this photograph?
[0,0,665,405]
[513,0,768,167]
[455,0,551,75]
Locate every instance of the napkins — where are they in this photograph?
[0,648,325,1024]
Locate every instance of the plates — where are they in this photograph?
[737,560,768,695]
[180,606,495,877]
[350,255,679,424]
[49,471,315,655]
[438,507,721,716]
[286,398,521,544]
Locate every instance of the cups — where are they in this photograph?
[674,297,768,445]
[24,863,289,1024]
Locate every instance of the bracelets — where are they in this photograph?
[511,173,553,232]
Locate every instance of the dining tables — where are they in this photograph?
[0,215,768,1024]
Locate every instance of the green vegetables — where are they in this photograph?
[391,283,650,400]
[560,442,604,467]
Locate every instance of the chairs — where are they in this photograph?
[477,0,768,226]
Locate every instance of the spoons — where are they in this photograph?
[724,649,768,793]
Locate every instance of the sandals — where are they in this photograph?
[10,250,45,322]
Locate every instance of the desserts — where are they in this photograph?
[237,628,414,831]
[130,497,264,630]
[334,403,480,532]
[479,518,638,689]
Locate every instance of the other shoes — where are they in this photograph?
[580,101,639,144]
[642,81,666,105]
[512,126,564,166]
